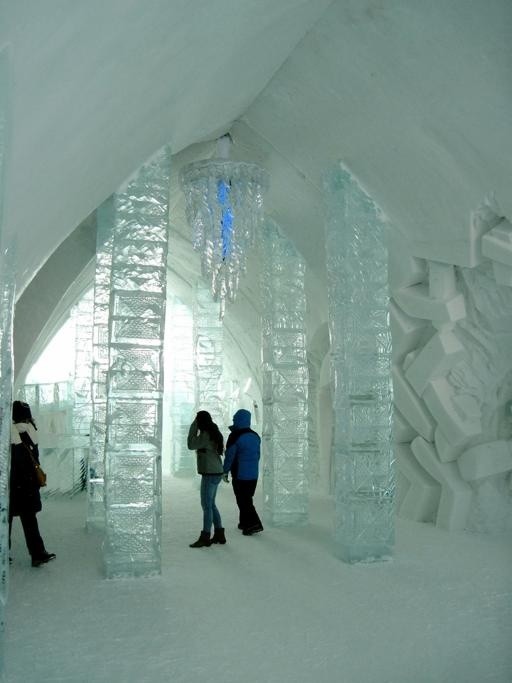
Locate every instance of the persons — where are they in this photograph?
[187,411,226,547]
[222,409,263,535]
[9,401,56,567]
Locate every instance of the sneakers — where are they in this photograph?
[238,523,263,535]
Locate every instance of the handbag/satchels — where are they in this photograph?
[36,464,46,487]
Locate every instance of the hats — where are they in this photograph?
[13,401,31,422]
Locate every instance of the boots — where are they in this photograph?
[32,544,56,566]
[189,528,226,547]
